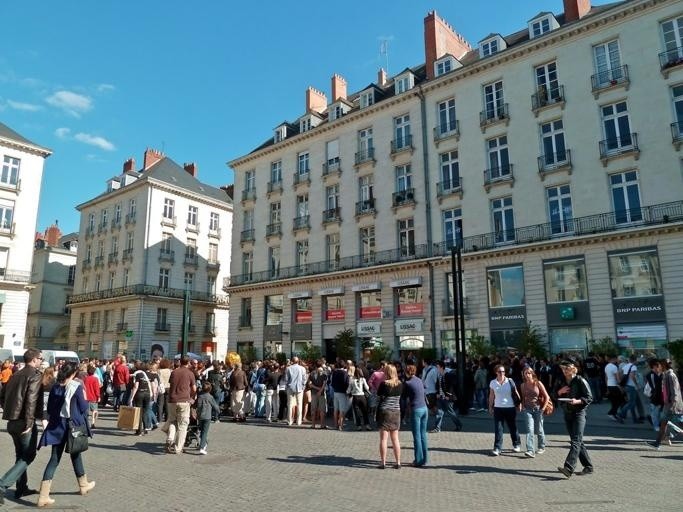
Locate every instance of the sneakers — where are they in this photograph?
[1,496,5,506]
[15,486,35,498]
[307,424,594,479]
[646,442,657,449]
[606,410,653,425]
[145,422,210,456]
[91,424,95,429]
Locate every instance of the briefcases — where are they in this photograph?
[118,405,140,430]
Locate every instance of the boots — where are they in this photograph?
[77,475,95,496]
[38,479,54,507]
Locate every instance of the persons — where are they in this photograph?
[127,360,158,437]
[0,346,45,506]
[583,352,601,404]
[473,361,488,412]
[174,353,306,367]
[230,362,249,423]
[253,361,270,418]
[36,362,96,506]
[162,356,196,455]
[41,366,54,433]
[0,363,12,412]
[604,354,622,421]
[519,367,550,457]
[262,363,283,424]
[345,367,372,430]
[150,343,165,361]
[71,365,87,401]
[113,356,131,412]
[488,364,522,455]
[284,357,308,426]
[549,358,593,478]
[402,364,429,466]
[614,355,643,424]
[142,364,160,431]
[158,359,171,422]
[428,361,462,433]
[246,363,257,416]
[367,361,390,431]
[330,360,349,430]
[192,382,220,455]
[321,349,583,367]
[10,360,19,374]
[99,368,113,408]
[208,365,223,423]
[420,357,446,427]
[306,359,327,429]
[393,361,407,425]
[55,358,120,368]
[375,363,405,469]
[82,365,101,428]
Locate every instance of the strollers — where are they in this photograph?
[182,397,207,452]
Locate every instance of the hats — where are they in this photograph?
[557,358,575,365]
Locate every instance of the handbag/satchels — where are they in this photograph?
[64,422,88,454]
[665,422,682,441]
[618,375,627,387]
[253,383,265,391]
[538,394,553,416]
[510,386,521,407]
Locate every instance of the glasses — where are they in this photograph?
[657,365,661,368]
[496,371,504,374]
[37,358,42,362]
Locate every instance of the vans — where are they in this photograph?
[0,349,29,370]
[44,350,80,377]
